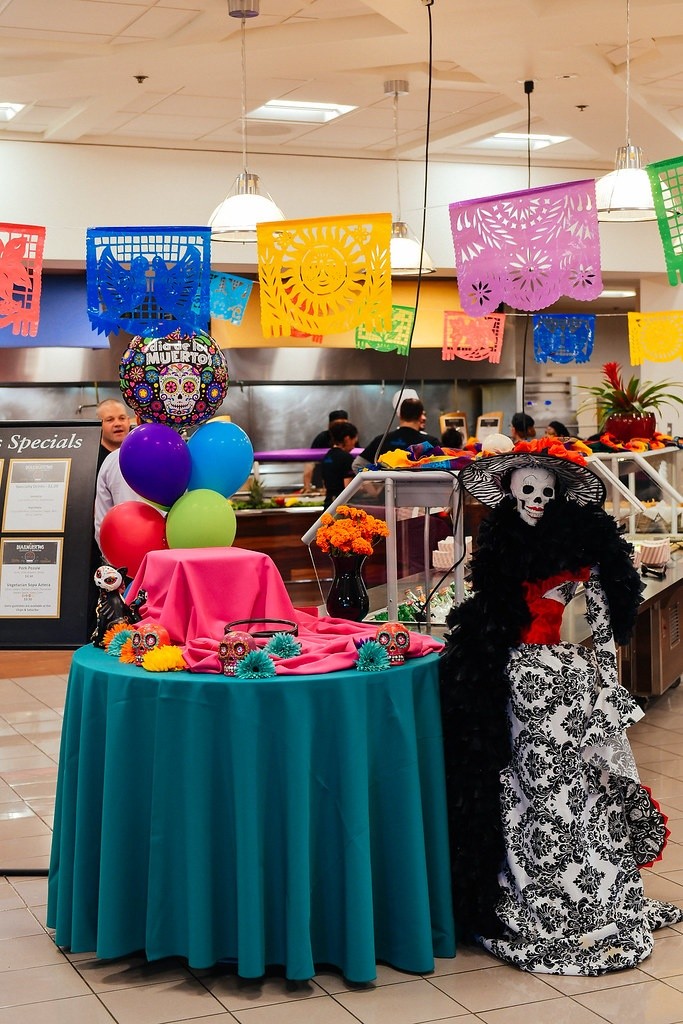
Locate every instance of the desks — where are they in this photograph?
[43,625,447,990]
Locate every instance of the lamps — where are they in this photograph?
[373,77,434,275]
[592,0,676,222]
[207,0,291,245]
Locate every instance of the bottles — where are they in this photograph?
[375,582,455,623]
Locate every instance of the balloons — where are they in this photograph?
[99,503,171,578]
[186,422,255,499]
[165,490,238,550]
[118,319,230,428]
[119,424,192,508]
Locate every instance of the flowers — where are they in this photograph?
[563,362,683,431]
[316,504,392,554]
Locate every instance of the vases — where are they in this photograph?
[605,411,654,440]
[325,550,372,622]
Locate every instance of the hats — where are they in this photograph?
[329,410,350,421]
[548,420,570,439]
[512,413,535,434]
[457,438,607,516]
[391,389,421,418]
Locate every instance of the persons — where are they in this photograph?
[95,400,130,475]
[300,411,360,494]
[439,427,463,451]
[353,403,443,473]
[544,421,570,437]
[508,413,536,442]
[311,422,359,513]
[94,448,167,560]
[442,438,683,974]
